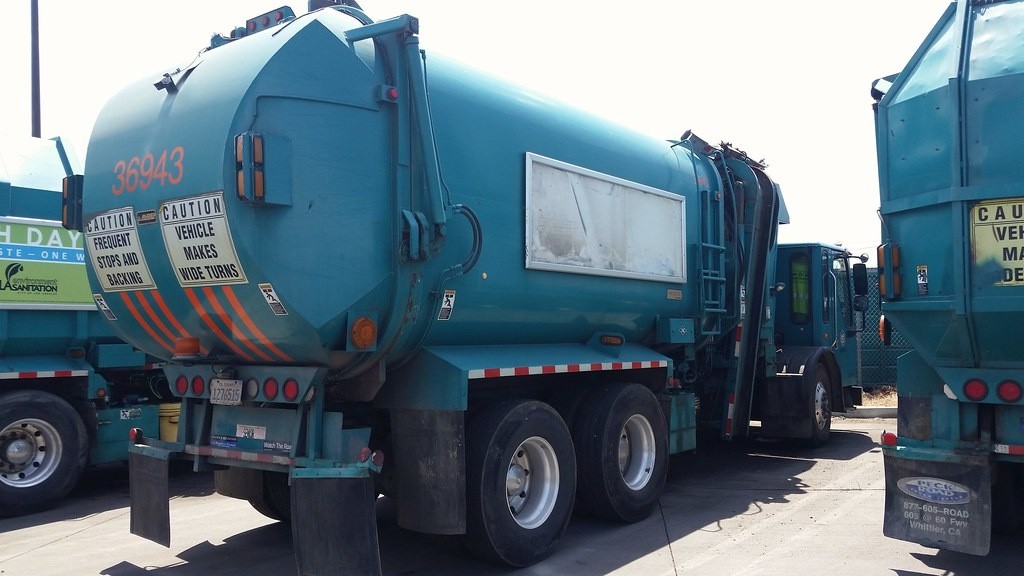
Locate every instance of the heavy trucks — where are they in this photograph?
[61,0,873,575]
[0,176,195,517]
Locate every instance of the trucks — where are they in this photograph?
[864,0,1023,560]
